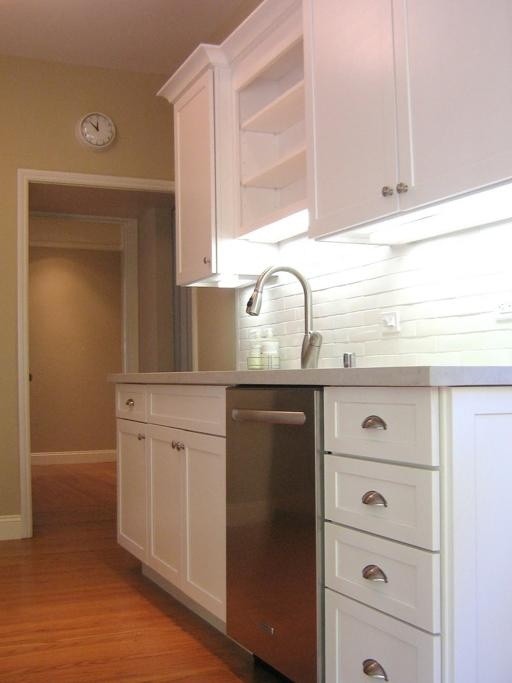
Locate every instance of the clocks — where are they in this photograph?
[76,112,116,148]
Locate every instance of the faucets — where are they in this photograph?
[245,265,323,369]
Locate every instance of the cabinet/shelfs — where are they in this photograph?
[323,386,512,683]
[303,0,512,239]
[114,383,226,623]
[174,69,278,286]
[236,5,307,238]
